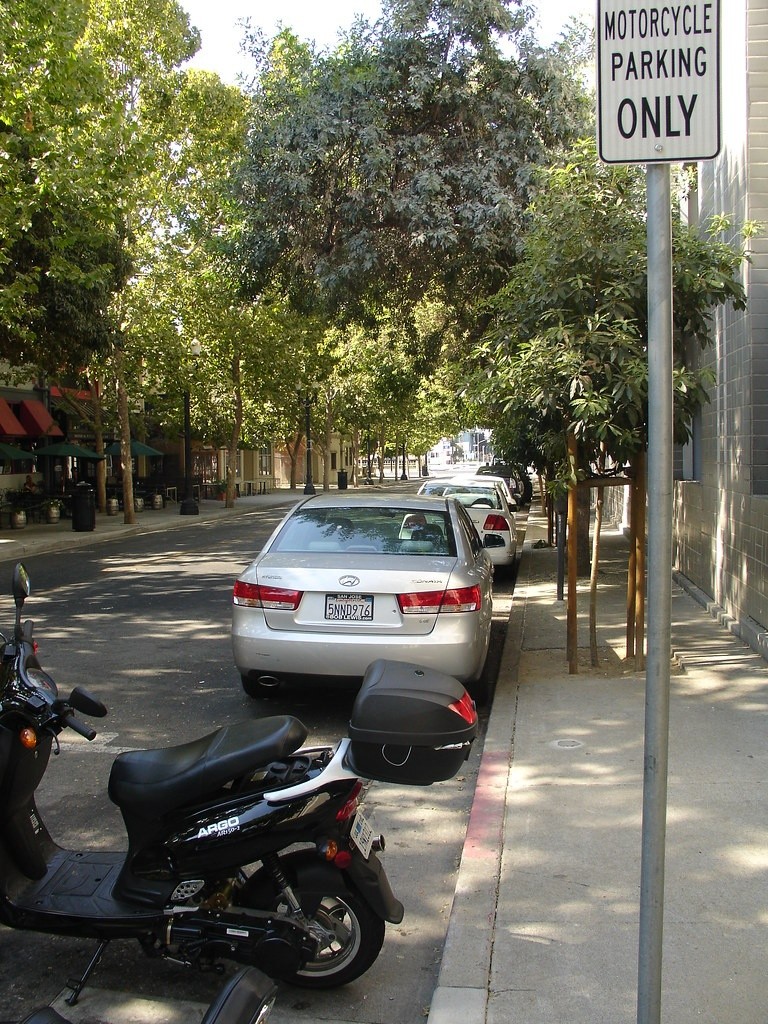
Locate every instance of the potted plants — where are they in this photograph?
[107,495,119,515]
[134,496,143,512]
[213,479,236,500]
[11,505,27,529]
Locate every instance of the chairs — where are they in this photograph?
[400,524,446,553]
[472,498,494,508]
[309,517,355,550]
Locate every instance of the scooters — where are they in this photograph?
[0,563,480,1007]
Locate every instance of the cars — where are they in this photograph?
[400,479,520,578]
[230,492,492,701]
[442,474,517,521]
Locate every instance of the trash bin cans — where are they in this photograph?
[72,484,96,532]
[337,469,348,489]
[422,466,428,476]
[362,466,367,477]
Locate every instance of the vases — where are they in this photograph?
[46,506,60,523]
[152,494,162,510]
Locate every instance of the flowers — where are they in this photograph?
[42,498,64,511]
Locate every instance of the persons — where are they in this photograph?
[24,475,36,494]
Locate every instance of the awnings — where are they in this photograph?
[0,399,27,438]
[105,439,164,458]
[0,445,38,459]
[32,443,98,458]
[19,400,63,436]
